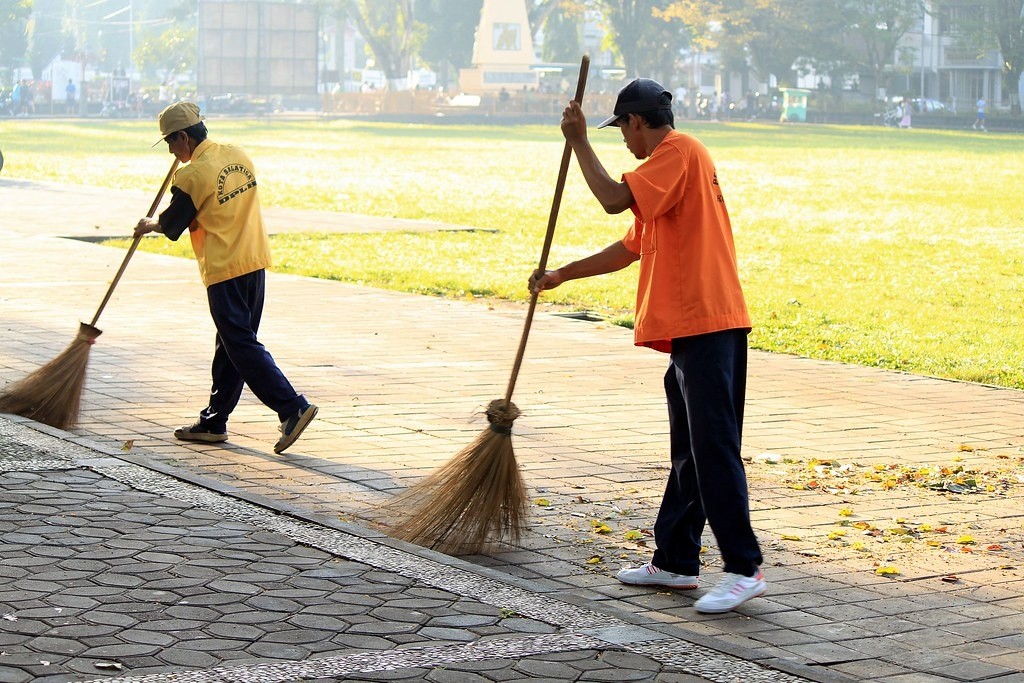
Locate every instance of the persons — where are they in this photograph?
[331,81,345,94]
[675,83,687,119]
[132,101,318,452]
[521,80,566,94]
[709,88,759,122]
[527,78,767,615]
[362,81,372,93]
[66,79,76,115]
[897,97,916,128]
[158,82,172,106]
[972,96,988,129]
[0,79,51,116]
[499,87,509,102]
[86,81,153,119]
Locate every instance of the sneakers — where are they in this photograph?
[617,562,699,589]
[174,420,228,443]
[693,571,768,612]
[274,404,318,453]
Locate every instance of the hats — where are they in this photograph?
[597,78,672,129]
[151,101,206,148]
